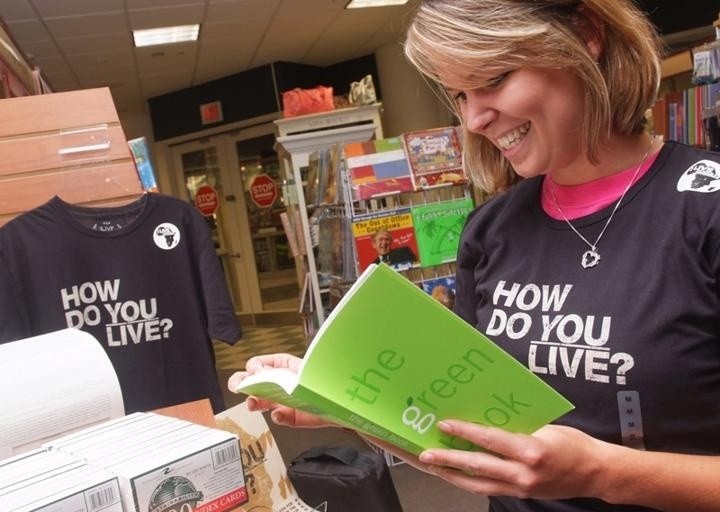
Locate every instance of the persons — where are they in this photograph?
[227,1,720,511]
[368,233,416,268]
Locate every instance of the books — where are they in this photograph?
[642,85,719,152]
[413,197,474,271]
[341,137,416,198]
[352,208,418,274]
[416,276,464,312]
[231,263,576,478]
[398,127,466,188]
[307,147,339,204]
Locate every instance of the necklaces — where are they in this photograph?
[547,135,654,268]
